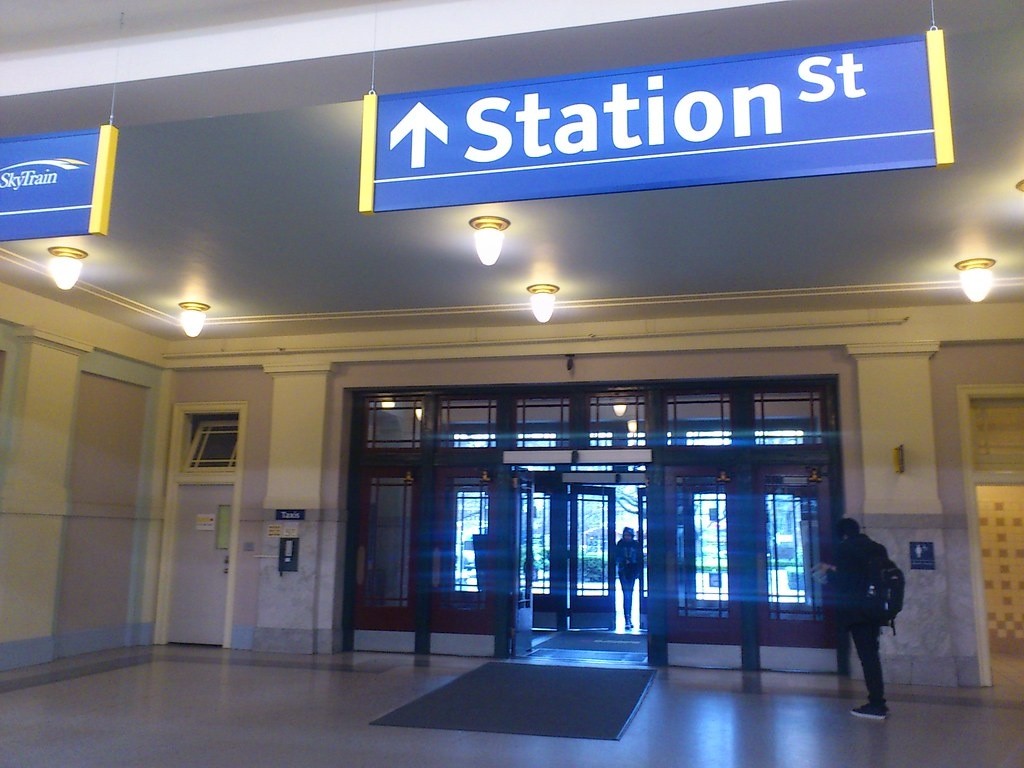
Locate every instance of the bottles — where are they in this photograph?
[868,585,875,597]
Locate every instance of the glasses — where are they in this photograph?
[624,533,632,536]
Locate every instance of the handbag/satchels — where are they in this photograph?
[625,563,642,579]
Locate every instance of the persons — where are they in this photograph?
[827,518,889,719]
[616,527,643,629]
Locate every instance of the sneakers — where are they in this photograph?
[851,697,886,719]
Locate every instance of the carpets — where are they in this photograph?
[368,661,658,742]
[530,631,648,654]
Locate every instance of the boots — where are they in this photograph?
[625,618,633,629]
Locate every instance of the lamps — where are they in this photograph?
[47,246,89,290]
[178,302,211,338]
[527,283,560,323]
[953,258,998,303]
[469,216,511,266]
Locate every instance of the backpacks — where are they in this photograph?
[839,541,905,636]
